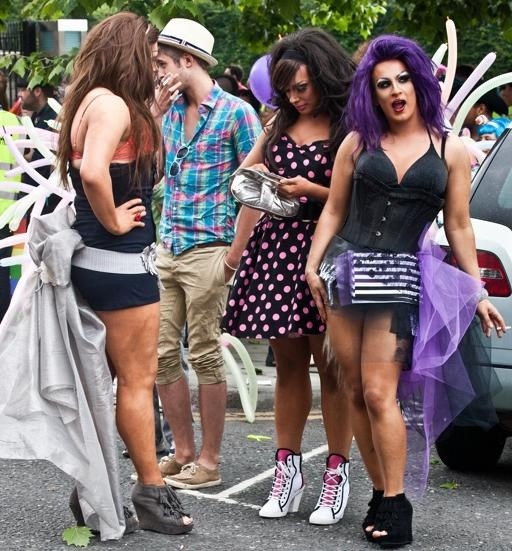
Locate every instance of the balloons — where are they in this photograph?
[248,54,280,110]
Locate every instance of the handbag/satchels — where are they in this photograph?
[337,251,424,309]
[231,167,300,218]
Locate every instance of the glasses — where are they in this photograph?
[169,146,188,177]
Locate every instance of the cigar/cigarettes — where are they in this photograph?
[496,326,512,331]
[162,74,173,85]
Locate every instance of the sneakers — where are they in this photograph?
[164,462,222,489]
[130,453,183,483]
[122,444,169,456]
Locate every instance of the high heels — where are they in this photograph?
[69,486,138,535]
[362,487,413,546]
[132,480,193,535]
[310,453,349,525]
[259,448,306,519]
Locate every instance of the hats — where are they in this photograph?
[17,69,55,87]
[158,18,219,67]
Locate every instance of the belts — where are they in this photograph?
[195,239,227,247]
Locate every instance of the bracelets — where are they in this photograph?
[224,258,237,271]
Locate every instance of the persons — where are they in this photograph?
[438,64,512,182]
[122,23,188,459]
[0,56,64,324]
[304,34,507,549]
[215,63,280,128]
[234,26,359,526]
[53,11,195,538]
[150,19,263,489]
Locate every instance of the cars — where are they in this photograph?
[435,117,511,475]
